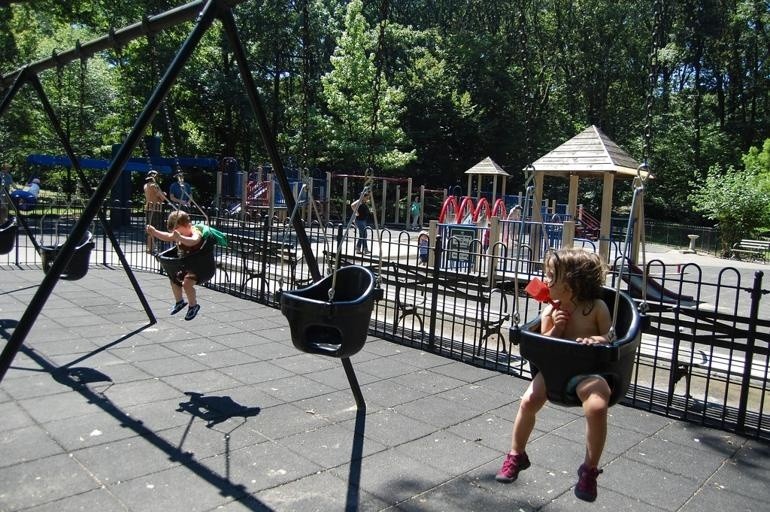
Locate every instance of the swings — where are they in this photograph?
[41,60,95,280]
[111,34,217,285]
[508,3,659,407]
[1,212,19,254]
[275,2,383,355]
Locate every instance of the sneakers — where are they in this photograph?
[573,460,605,505]
[183,304,202,322]
[355,246,371,256]
[493,449,534,485]
[168,299,189,317]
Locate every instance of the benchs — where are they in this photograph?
[730,239,770,264]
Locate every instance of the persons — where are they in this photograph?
[482,223,491,259]
[143,169,169,256]
[146,210,207,321]
[350,191,373,255]
[0,163,21,204]
[170,168,194,214]
[415,230,430,266]
[26,178,41,200]
[410,196,422,230]
[1,205,9,226]
[493,247,617,503]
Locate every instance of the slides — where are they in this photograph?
[230,180,268,217]
[612,259,706,307]
